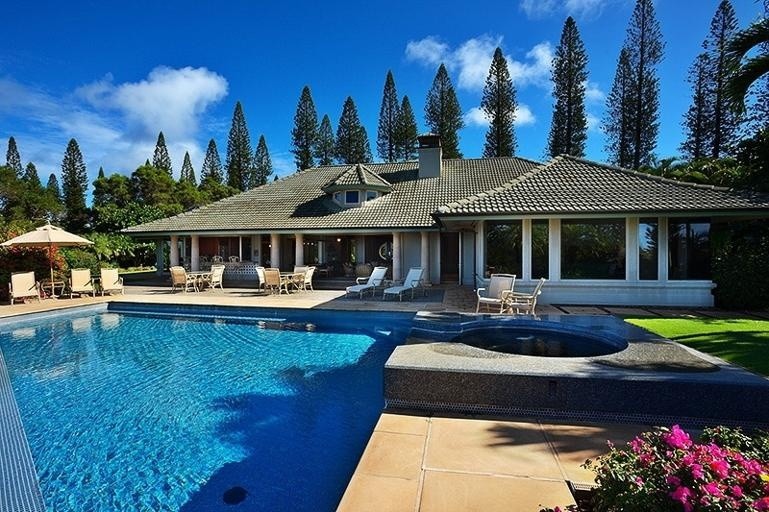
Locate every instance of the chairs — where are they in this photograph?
[501,276,547,313]
[166,248,434,304]
[9,265,125,306]
[475,271,518,312]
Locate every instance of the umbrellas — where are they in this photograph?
[0,220,96,299]
